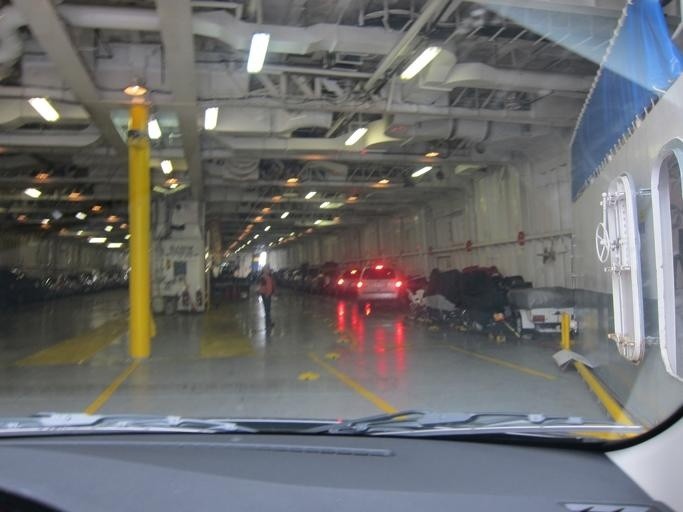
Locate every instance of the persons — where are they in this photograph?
[255,263,275,327]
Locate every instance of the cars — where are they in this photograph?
[271,259,412,314]
[0,261,128,307]
